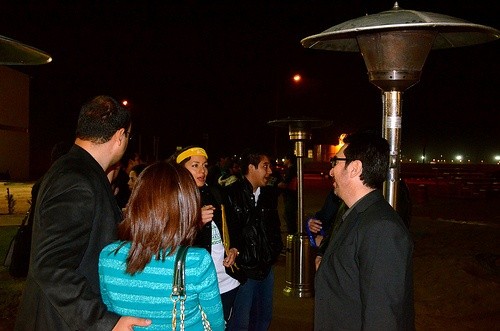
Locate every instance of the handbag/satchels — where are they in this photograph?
[3,201,39,279]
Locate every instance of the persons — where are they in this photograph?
[314,133,414,331]
[99,160,227,331]
[107,138,152,212]
[305,186,349,251]
[278,153,298,234]
[224,154,283,331]
[15,96,151,331]
[206,146,285,213]
[176,145,247,331]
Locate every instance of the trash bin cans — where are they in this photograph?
[291,232,315,299]
[282,235,295,298]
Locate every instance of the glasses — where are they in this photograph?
[330,156,353,169]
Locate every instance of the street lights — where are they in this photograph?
[300,0,500,214]
[265,116,331,299]
